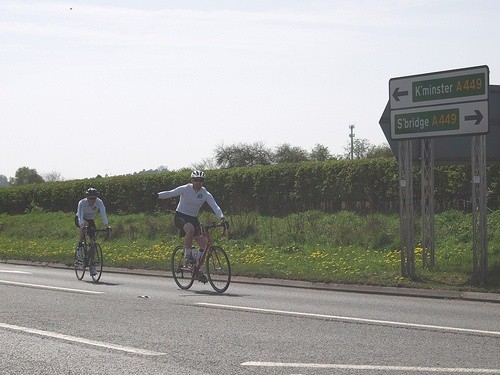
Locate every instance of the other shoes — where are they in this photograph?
[184,254,197,265]
[76,246,83,258]
[196,271,207,283]
[91,266,98,275]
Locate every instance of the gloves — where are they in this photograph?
[220,218,230,230]
[151,191,159,200]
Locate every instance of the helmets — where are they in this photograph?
[191,170,206,179]
[85,187,98,196]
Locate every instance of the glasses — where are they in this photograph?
[192,178,204,182]
[88,197,97,200]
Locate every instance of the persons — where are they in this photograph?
[151,170,229,283]
[75,188,109,276]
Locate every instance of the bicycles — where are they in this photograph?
[170,220,232,293]
[74,225,112,283]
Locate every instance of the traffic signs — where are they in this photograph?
[389,100,489,141]
[388,65,489,110]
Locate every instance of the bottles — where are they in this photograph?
[198,249,204,261]
[87,244,91,251]
[191,247,196,260]
